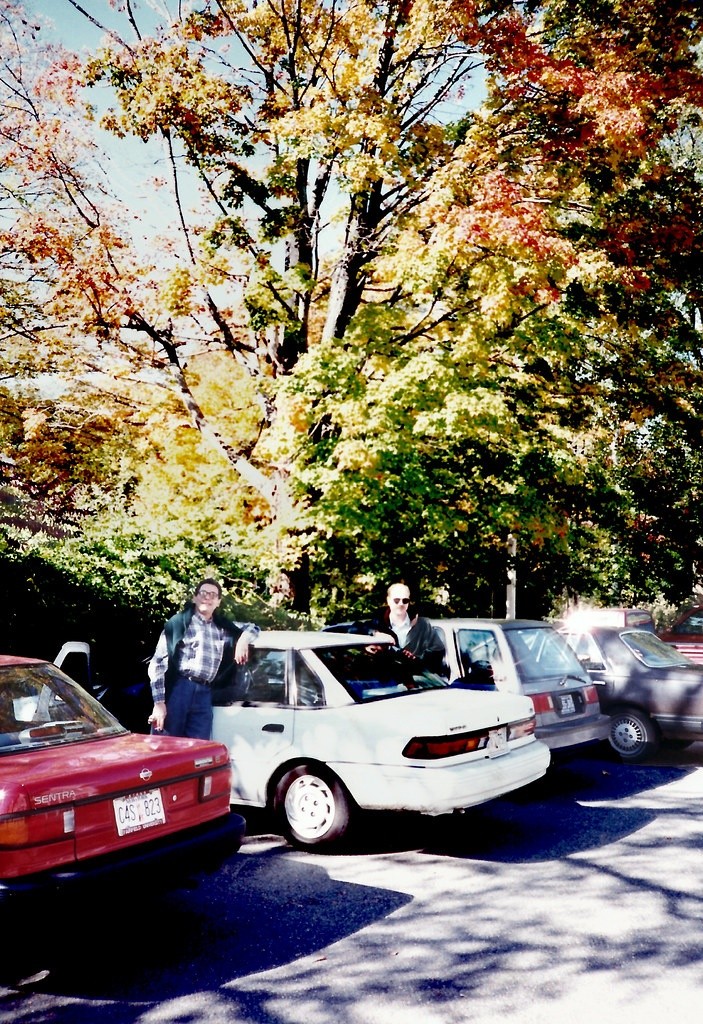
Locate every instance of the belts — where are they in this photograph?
[179,672,209,686]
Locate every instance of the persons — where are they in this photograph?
[148,578,260,741]
[348,583,446,684]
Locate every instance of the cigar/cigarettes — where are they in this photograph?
[155,727,161,730]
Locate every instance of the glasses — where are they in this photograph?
[198,588,219,599]
[394,597,409,604]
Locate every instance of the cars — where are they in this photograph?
[322,619,611,748]
[37,628,552,849]
[553,607,703,764]
[0,654,246,925]
